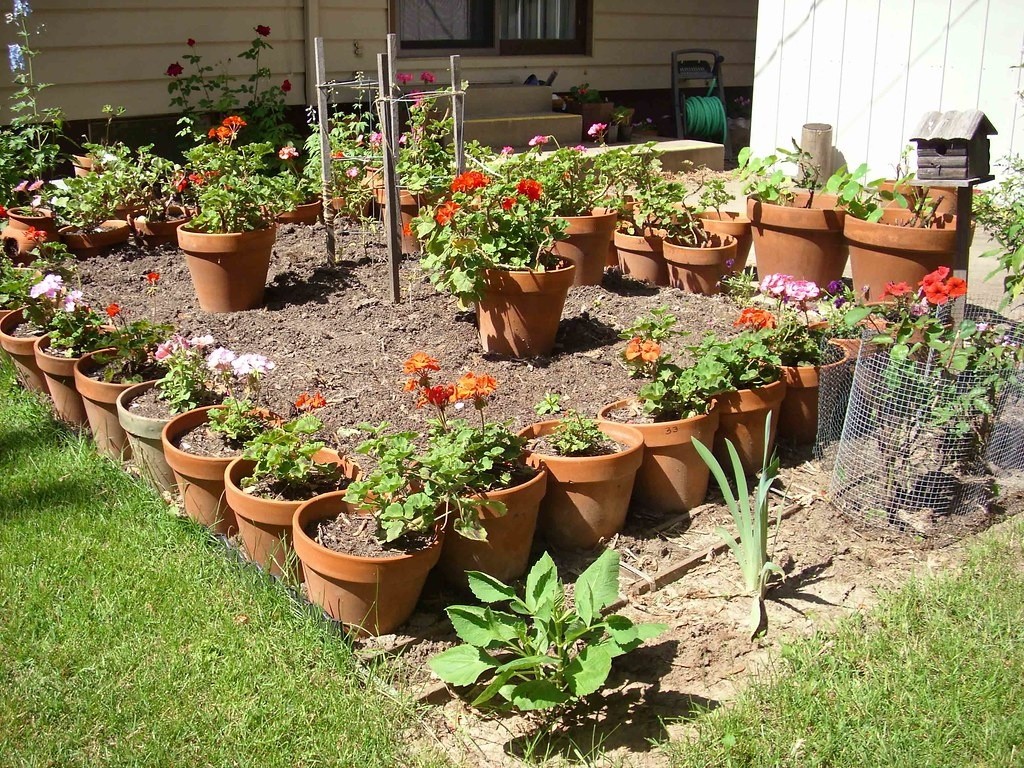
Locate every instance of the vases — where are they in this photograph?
[542,207,617,286]
[474,254,575,358]
[364,166,384,217]
[878,178,921,215]
[714,362,787,479]
[870,405,988,532]
[861,301,954,385]
[0,301,91,396]
[745,336,850,443]
[126,205,198,246]
[807,320,887,411]
[57,220,128,257]
[516,418,642,550]
[693,212,752,276]
[291,490,443,636]
[662,229,737,296]
[372,188,441,257]
[602,126,617,143]
[108,182,162,227]
[271,197,321,229]
[223,447,362,583]
[596,395,719,511]
[592,191,641,270]
[843,209,980,309]
[0,207,59,266]
[176,222,275,312]
[924,181,958,216]
[33,325,129,430]
[161,405,288,536]
[633,201,697,242]
[576,103,614,139]
[318,194,368,225]
[71,152,97,178]
[73,348,154,459]
[613,228,672,285]
[116,379,230,496]
[619,126,632,141]
[406,447,546,588]
[746,192,849,297]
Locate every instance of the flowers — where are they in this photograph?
[0,0,1011,552]
